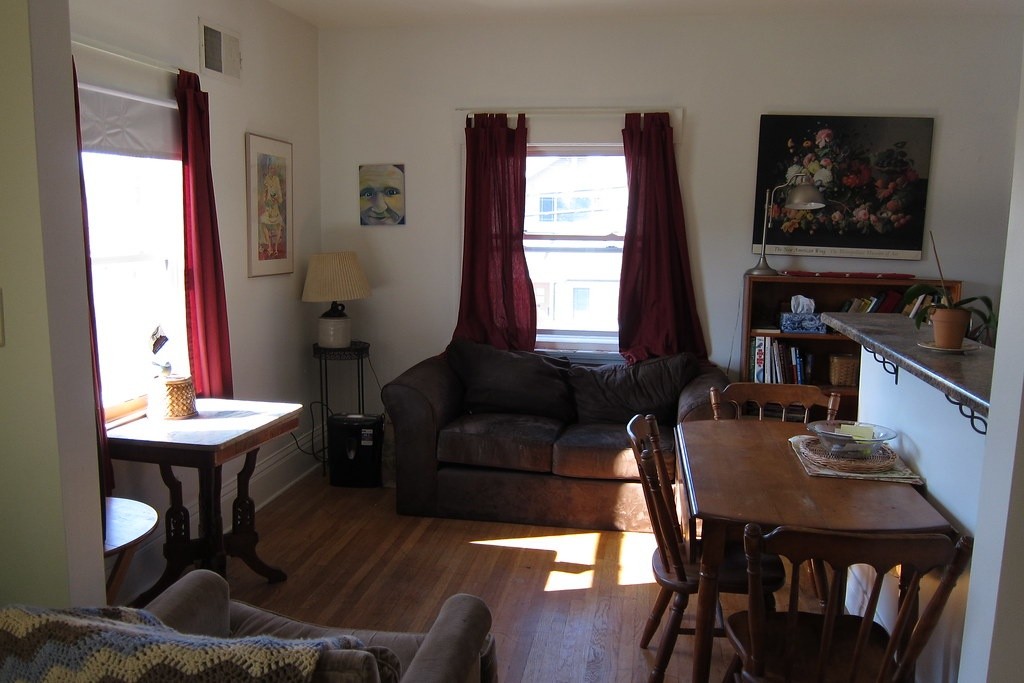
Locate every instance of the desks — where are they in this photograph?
[672,419,961,683]
[103,496,159,603]
[111,397,304,585]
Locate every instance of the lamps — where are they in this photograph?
[746,172,826,276]
[299,251,373,347]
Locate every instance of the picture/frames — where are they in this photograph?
[247,131,295,277]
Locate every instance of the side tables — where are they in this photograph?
[312,341,372,478]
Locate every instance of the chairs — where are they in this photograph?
[711,381,841,418]
[626,416,786,683]
[722,524,980,683]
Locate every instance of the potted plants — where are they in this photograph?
[904,285,1001,351]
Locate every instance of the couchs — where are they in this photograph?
[377,337,732,528]
[0,571,501,683]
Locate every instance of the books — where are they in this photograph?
[751,320,780,333]
[750,336,813,385]
[901,294,948,326]
[839,292,900,314]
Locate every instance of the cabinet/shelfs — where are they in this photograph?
[738,273,966,416]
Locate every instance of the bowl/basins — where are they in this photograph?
[807,421,897,459]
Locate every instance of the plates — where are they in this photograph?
[916,340,980,354]
